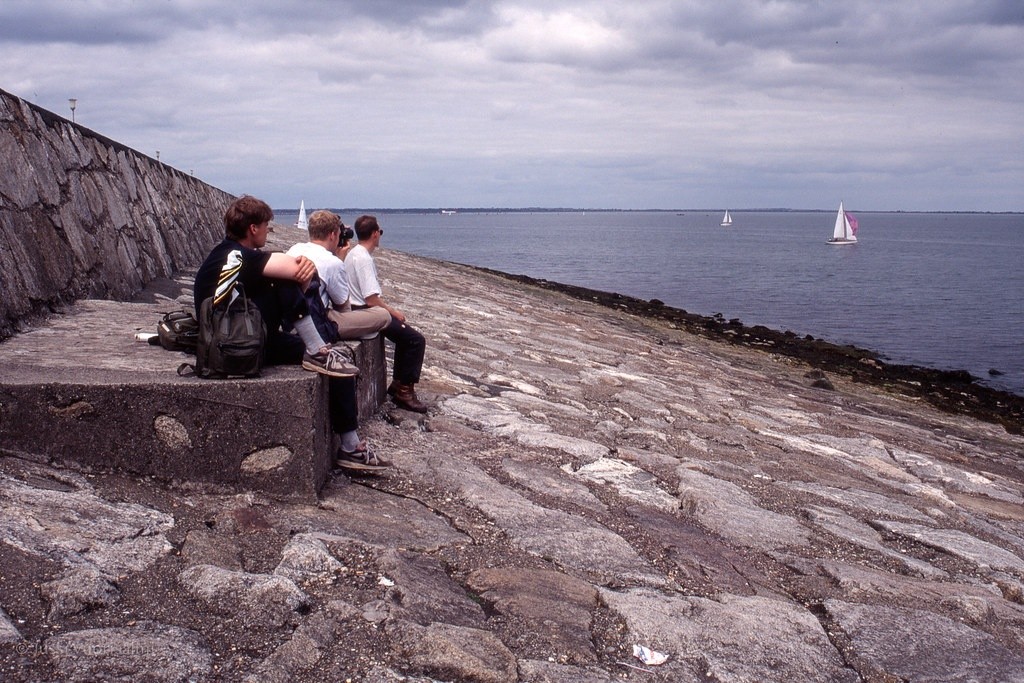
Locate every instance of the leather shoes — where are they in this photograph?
[387,380,428,414]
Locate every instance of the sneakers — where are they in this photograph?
[336,440,393,470]
[302,343,361,377]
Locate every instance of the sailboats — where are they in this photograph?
[719,207,734,227]
[825,199,858,245]
[294,199,310,232]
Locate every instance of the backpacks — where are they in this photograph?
[148,310,200,354]
[177,281,268,380]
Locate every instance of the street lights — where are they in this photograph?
[156,150,160,160]
[69,97,77,121]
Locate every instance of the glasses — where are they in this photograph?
[378,230,383,235]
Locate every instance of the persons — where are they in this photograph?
[286,209,426,412]
[194,195,393,470]
